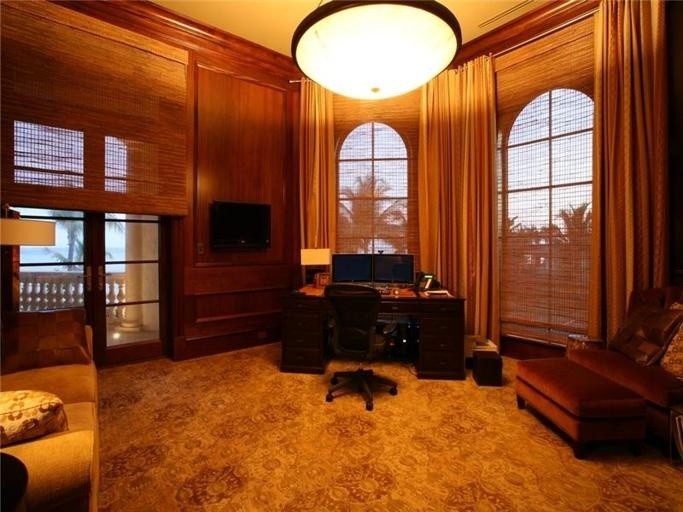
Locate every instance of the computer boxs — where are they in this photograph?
[396,323,415,363]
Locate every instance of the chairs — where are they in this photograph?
[321,284,400,411]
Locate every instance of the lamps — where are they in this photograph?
[300,246,331,288]
[290,0,464,101]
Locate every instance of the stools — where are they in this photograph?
[471,350,503,386]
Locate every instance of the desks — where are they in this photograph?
[280,279,467,381]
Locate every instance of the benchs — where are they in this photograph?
[0,302,102,511]
[513,355,646,460]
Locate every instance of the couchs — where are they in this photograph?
[569,289,683,473]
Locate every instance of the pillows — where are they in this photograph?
[0,388,70,447]
[0,305,92,376]
[607,295,683,380]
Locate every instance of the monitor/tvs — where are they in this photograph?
[210,200,271,250]
[373,254,415,283]
[332,254,373,286]
